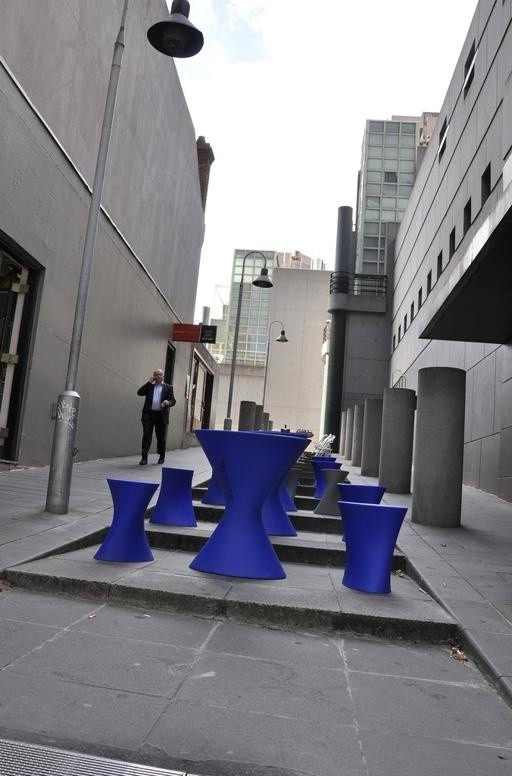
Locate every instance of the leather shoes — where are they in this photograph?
[158,457,164,464]
[140,459,148,465]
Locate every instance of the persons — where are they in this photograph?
[136,368,177,465]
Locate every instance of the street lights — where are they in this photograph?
[46,1,204,516]
[224,251,274,432]
[263,321,287,407]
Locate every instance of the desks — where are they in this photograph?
[190,429,312,580]
